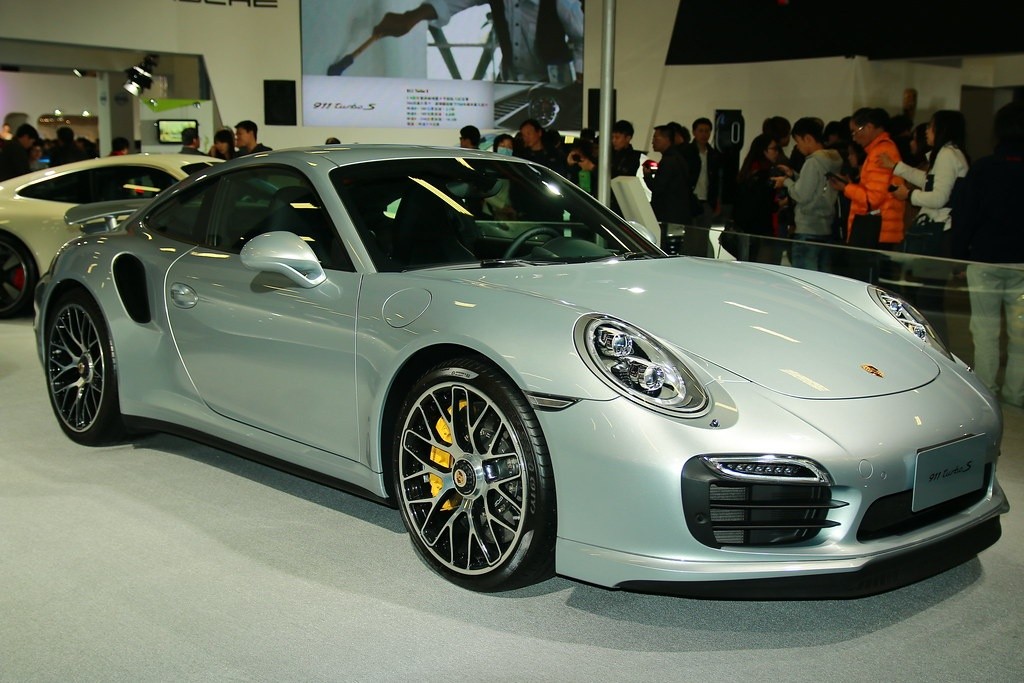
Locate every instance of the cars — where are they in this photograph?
[0,150,279,320]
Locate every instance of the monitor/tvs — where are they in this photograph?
[156,119,198,144]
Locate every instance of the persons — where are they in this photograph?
[109,137,129,155]
[737,107,969,313]
[566,118,719,258]
[0,121,98,182]
[373,0,583,84]
[460,126,568,220]
[949,103,1024,407]
[208,120,273,160]
[179,127,204,154]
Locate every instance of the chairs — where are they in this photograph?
[396,181,485,268]
[268,187,345,270]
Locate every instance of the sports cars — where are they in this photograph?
[35,144,1010,594]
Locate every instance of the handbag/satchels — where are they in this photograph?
[848,192,882,249]
[719,223,756,262]
[904,214,945,257]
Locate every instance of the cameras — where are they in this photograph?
[768,167,786,189]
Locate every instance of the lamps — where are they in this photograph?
[150,98,158,107]
[194,102,201,108]
[121,53,159,96]
[72,68,88,78]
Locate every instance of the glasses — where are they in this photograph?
[926,125,934,129]
[851,126,865,135]
[767,145,778,151]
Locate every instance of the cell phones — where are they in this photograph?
[888,184,898,192]
[823,172,848,186]
[572,154,582,163]
